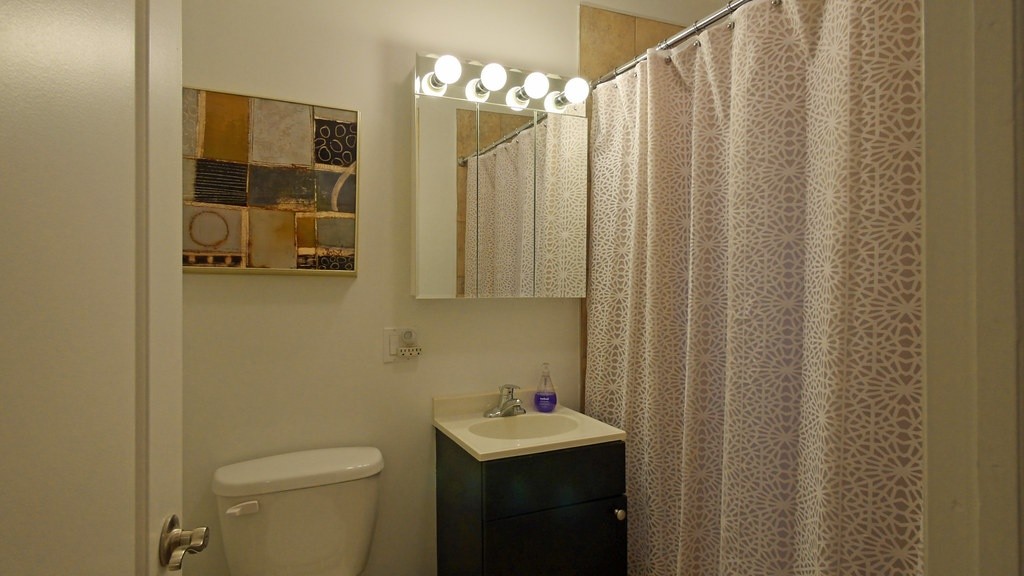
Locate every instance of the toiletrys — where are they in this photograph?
[535,358,557,416]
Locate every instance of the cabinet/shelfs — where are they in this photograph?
[435,426,629,576]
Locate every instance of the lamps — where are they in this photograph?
[515,72,550,104]
[554,77,590,108]
[430,54,463,89]
[474,62,507,96]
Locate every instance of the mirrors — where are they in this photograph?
[415,97,587,301]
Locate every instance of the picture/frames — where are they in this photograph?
[182,86,364,277]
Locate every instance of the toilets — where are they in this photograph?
[209,445,385,575]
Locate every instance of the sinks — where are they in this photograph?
[432,388,629,462]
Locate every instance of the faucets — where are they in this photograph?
[491,382,527,420]
